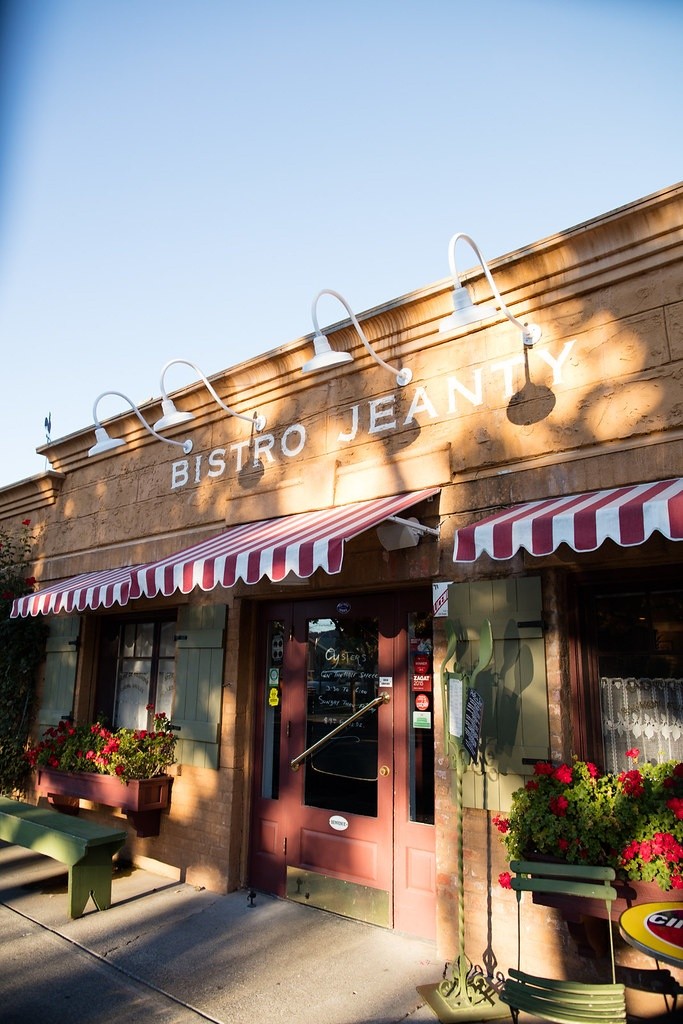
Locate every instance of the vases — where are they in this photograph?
[523,848,683,924]
[34,762,174,812]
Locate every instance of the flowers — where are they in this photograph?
[491,747,683,891]
[19,701,180,786]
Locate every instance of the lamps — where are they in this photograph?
[301,288,414,387]
[439,231,542,345]
[87,391,194,458]
[152,360,266,432]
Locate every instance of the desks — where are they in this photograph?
[618,901,683,1024]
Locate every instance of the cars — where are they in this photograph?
[308,669,365,697]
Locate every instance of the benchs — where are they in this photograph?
[0,796,128,920]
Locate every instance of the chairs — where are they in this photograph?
[499,860,626,1024]
